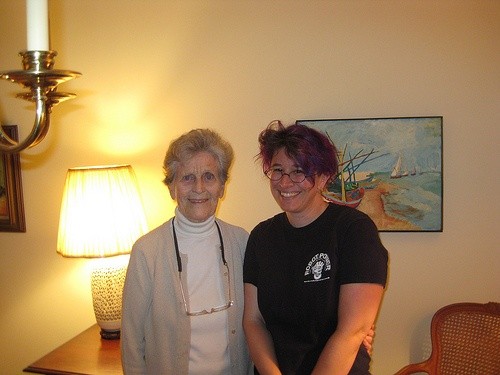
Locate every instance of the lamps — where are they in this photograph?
[55,165,150,339]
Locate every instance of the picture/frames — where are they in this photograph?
[0,125,27,233]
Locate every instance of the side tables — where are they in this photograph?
[23,323,124,375]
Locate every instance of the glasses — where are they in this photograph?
[264,167,321,184]
[178,264,234,316]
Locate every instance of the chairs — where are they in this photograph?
[383,302,500,375]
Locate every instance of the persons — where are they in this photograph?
[120,129,379,375]
[241,120,390,375]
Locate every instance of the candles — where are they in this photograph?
[26,0,49,51]
